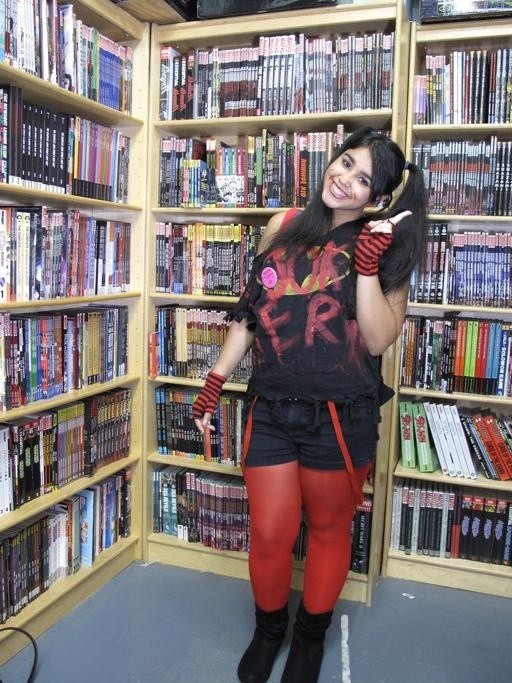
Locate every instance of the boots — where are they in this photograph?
[280,599,332,683]
[237,600,289,683]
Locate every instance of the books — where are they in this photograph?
[2,1,132,614]
[152,33,397,572]
[391,44,511,566]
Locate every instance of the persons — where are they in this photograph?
[191,130,429,681]
